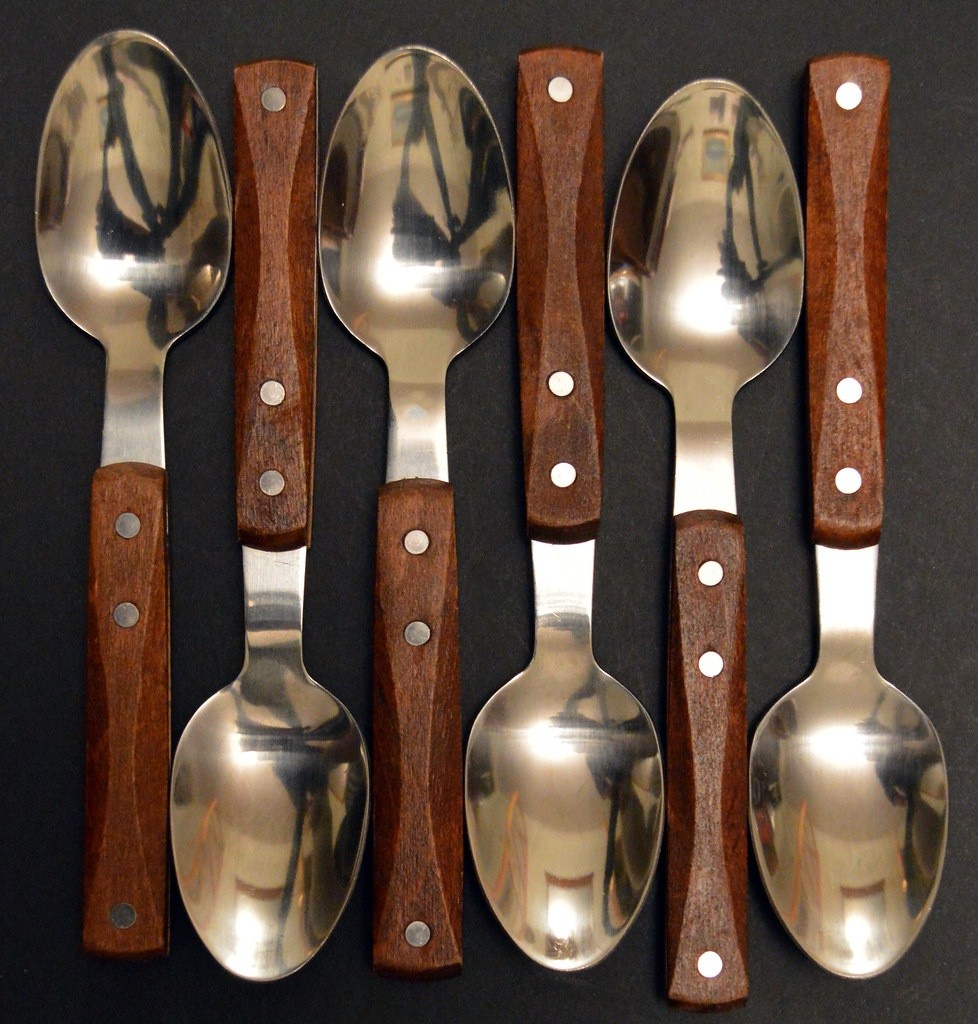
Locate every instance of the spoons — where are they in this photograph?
[173,60,368,984]
[316,47,514,963]
[464,49,664,976]
[606,79,806,1002]
[738,53,947,976]
[38,32,231,956]
